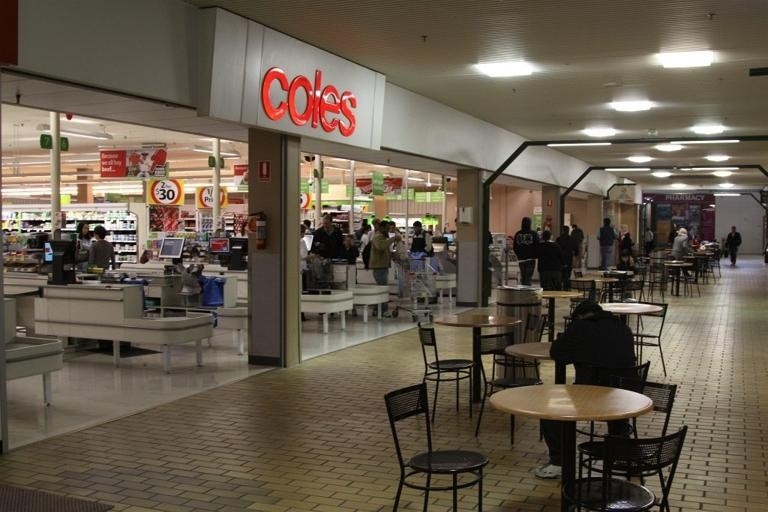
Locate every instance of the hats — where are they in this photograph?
[676,228,688,236]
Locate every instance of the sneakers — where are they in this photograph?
[534,464,562,480]
[383,311,392,318]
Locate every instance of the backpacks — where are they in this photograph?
[363,240,373,270]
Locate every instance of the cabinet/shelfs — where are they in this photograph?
[1,209,139,275]
[180,210,247,240]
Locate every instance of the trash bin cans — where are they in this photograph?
[650,253,668,291]
[498,285,544,378]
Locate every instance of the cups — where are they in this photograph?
[104,221,138,262]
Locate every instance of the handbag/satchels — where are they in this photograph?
[724,250,729,257]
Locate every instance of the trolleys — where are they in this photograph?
[391,256,440,324]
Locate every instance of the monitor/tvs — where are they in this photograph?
[158,237,186,258]
[48,239,76,265]
[442,233,454,242]
[432,237,448,250]
[229,237,248,256]
[302,234,315,251]
[209,236,230,254]
[43,241,54,263]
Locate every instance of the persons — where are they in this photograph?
[667,224,701,279]
[513,217,584,291]
[723,224,742,267]
[74,220,94,254]
[300,209,434,319]
[86,224,116,271]
[641,224,654,255]
[533,299,642,480]
[596,217,640,298]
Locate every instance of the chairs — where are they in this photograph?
[569,242,727,313]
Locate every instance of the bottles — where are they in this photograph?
[176,232,197,244]
